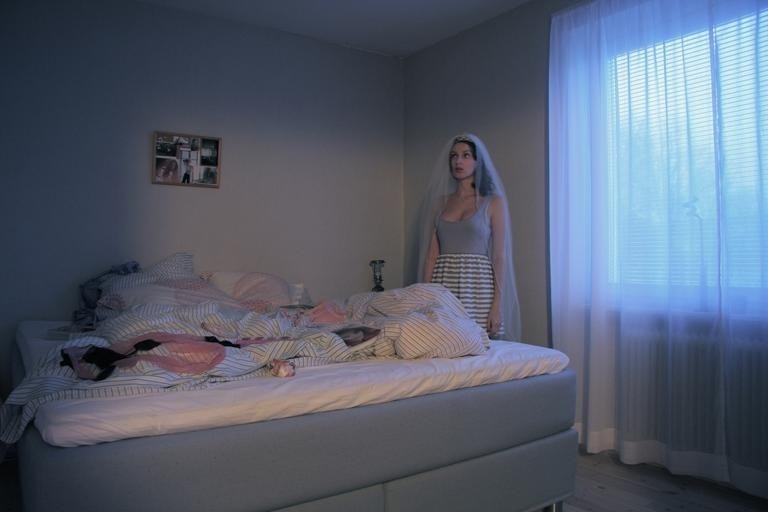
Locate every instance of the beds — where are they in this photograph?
[9,318,579,510]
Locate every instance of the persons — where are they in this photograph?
[334,327,372,346]
[181,160,194,184]
[200,167,216,179]
[156,159,179,178]
[412,133,524,343]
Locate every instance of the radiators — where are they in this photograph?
[616,327,768,489]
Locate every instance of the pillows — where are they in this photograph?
[77,260,148,308]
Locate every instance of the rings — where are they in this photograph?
[495,320,498,324]
[498,320,501,324]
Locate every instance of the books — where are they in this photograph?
[322,318,381,352]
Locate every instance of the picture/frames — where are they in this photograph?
[150,129,222,190]
